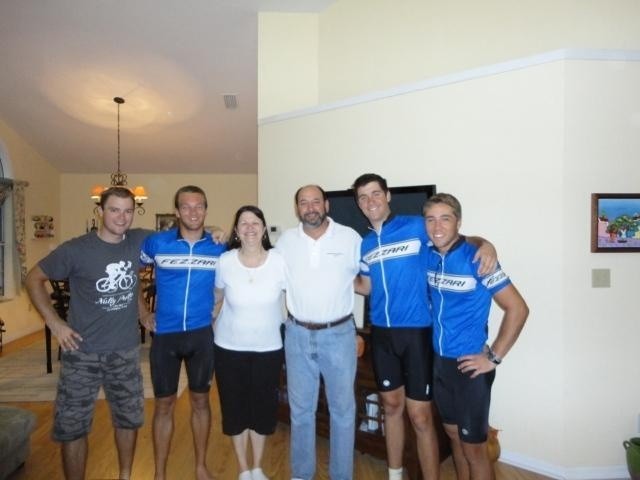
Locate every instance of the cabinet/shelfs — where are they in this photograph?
[275,354,453,480]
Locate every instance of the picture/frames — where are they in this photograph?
[590,193,640,253]
[155,213,180,238]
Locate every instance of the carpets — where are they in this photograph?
[1,337,188,404]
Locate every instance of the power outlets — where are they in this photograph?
[591,268,611,288]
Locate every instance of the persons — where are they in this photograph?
[138,184,229,480]
[422,193,531,480]
[274,184,365,480]
[210,205,288,480]
[23,186,229,480]
[352,173,499,480]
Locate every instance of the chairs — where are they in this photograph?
[46,265,157,373]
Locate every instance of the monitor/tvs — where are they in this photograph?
[324,184,436,240]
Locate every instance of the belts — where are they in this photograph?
[287,311,354,330]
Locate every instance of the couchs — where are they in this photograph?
[0,407,38,480]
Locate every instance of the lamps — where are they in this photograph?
[90,96,147,215]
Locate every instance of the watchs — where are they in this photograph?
[485,350,502,364]
